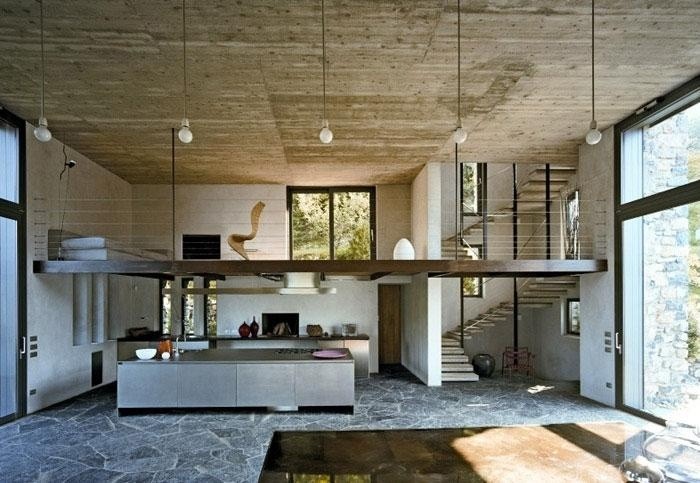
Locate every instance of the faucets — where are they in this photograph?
[175,337,180,356]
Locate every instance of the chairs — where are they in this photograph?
[503,347,536,383]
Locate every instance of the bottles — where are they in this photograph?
[159,336,172,353]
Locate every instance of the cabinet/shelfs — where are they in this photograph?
[116,358,355,417]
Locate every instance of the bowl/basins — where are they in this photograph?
[136,348,157,360]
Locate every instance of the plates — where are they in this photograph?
[312,351,348,358]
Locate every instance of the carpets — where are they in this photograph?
[257,419,686,483]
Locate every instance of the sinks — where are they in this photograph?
[172,348,205,353]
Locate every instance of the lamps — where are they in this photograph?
[33,1,53,141]
[585,0,602,145]
[453,0,468,144]
[319,0,333,143]
[178,0,193,142]
